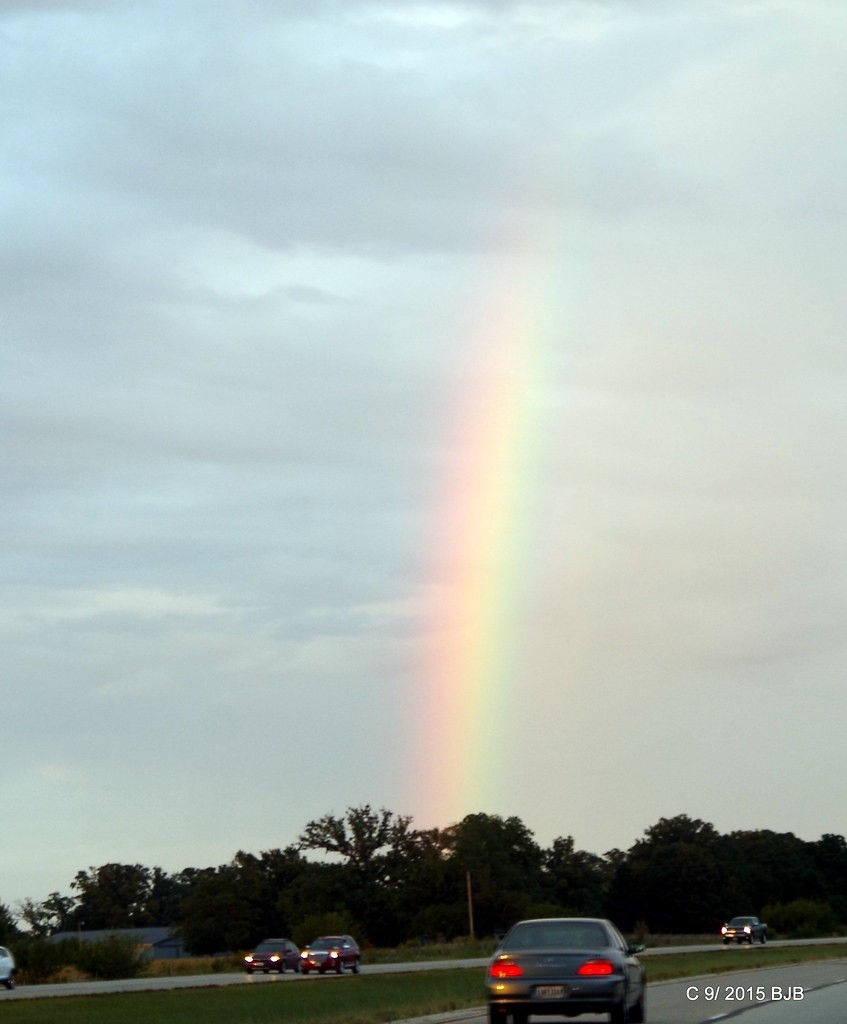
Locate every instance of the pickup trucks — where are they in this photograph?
[721,916,769,945]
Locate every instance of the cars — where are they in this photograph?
[244,938,302,974]
[484,917,647,1024]
[0,946,18,989]
[301,935,360,974]
